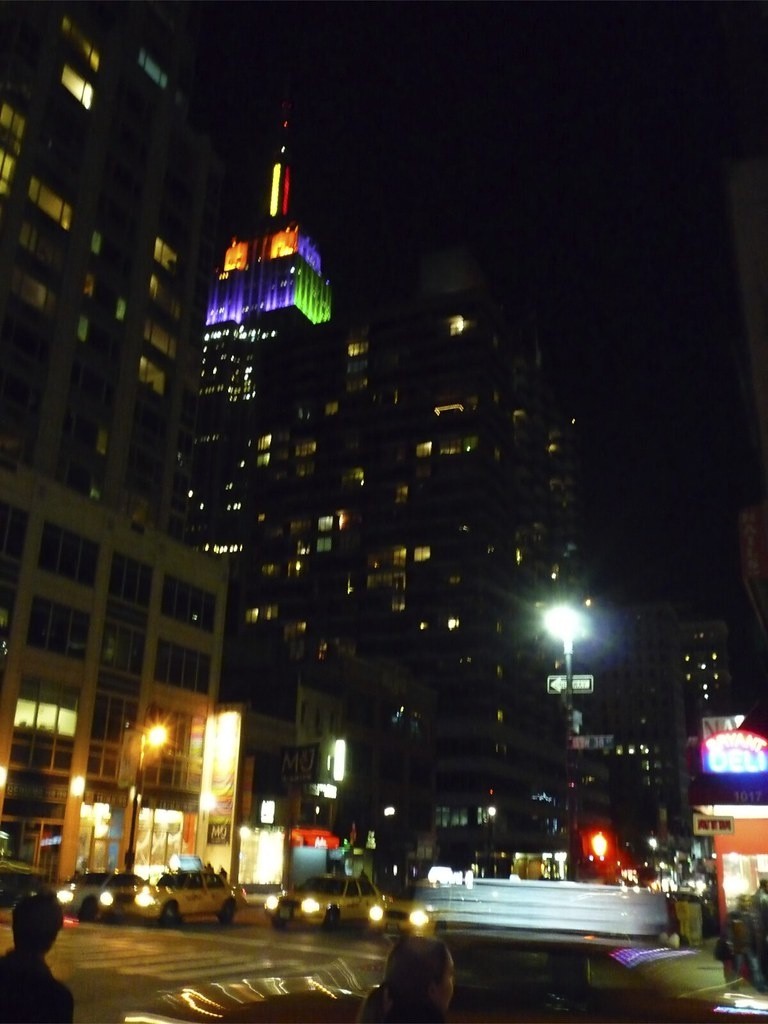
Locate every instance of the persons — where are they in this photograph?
[358,937,454,1024]
[0,890,74,1024]
[220,868,227,879]
[206,864,214,873]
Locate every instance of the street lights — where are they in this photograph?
[547,606,584,882]
[120,724,170,874]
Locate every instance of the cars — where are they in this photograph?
[57,871,153,925]
[270,872,386,934]
[129,869,237,927]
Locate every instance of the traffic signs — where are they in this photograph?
[547,674,594,695]
